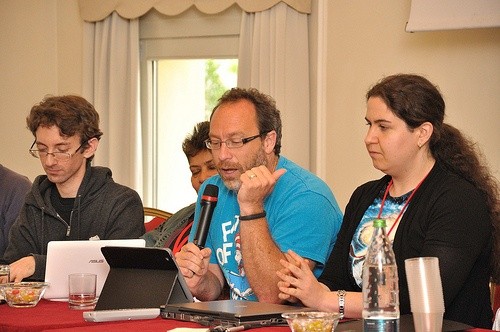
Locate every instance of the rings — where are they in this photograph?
[250,174,256,179]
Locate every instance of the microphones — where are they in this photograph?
[194,184,219,250]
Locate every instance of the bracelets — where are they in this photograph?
[238,211,266,220]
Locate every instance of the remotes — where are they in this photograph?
[83,308,160,322]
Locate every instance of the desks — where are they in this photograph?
[0,301,500,332]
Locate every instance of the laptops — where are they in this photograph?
[43,238,145,302]
[159,300,315,329]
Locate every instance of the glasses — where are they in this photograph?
[204,134,261,150]
[29,139,86,160]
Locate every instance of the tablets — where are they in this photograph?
[101,245,195,305]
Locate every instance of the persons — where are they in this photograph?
[0,164,33,258]
[139,121,219,256]
[175,88,343,305]
[0,95,146,284]
[277,74,500,330]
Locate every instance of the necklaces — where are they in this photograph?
[377,180,423,237]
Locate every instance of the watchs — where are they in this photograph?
[337,289,346,319]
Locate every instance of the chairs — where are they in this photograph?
[143,207,173,234]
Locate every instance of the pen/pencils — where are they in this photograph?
[225,324,262,332]
